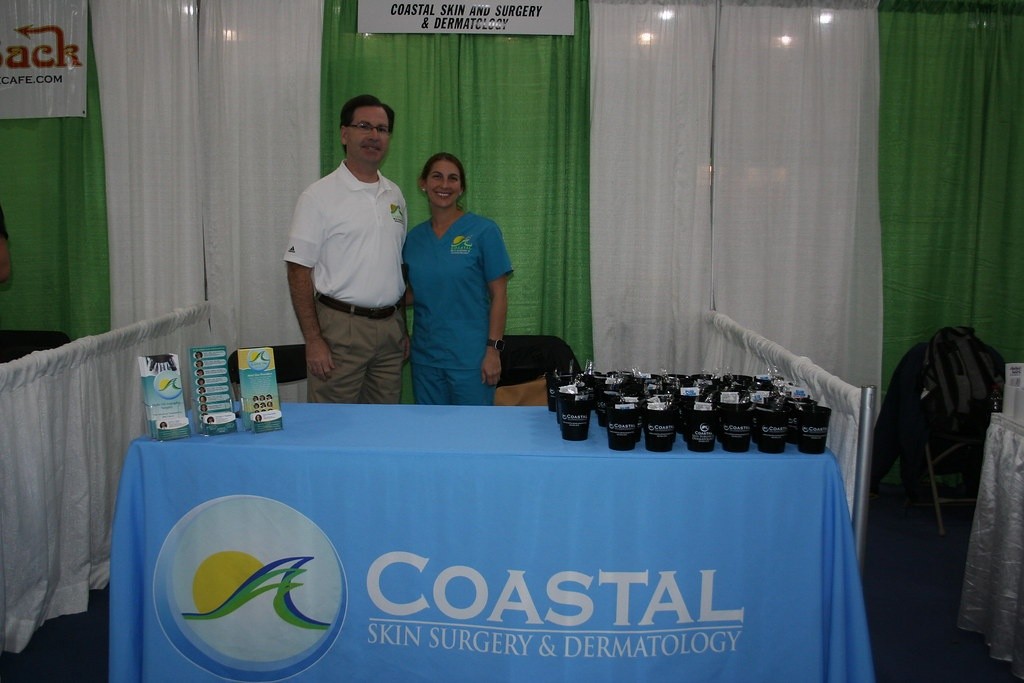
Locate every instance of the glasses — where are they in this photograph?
[344,121,392,137]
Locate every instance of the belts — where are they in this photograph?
[316,292,403,320]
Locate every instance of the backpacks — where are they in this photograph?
[918,324,1007,439]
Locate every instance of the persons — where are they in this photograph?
[402,152,514,406]
[0,204,10,283]
[283,94,409,404]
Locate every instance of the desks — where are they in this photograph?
[957,413,1024,683]
[109,403,877,683]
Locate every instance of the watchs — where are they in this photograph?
[487,339,505,352]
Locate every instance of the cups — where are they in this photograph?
[546,370,832,454]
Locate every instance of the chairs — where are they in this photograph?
[900,335,993,536]
[228,343,308,401]
[496,335,581,406]
